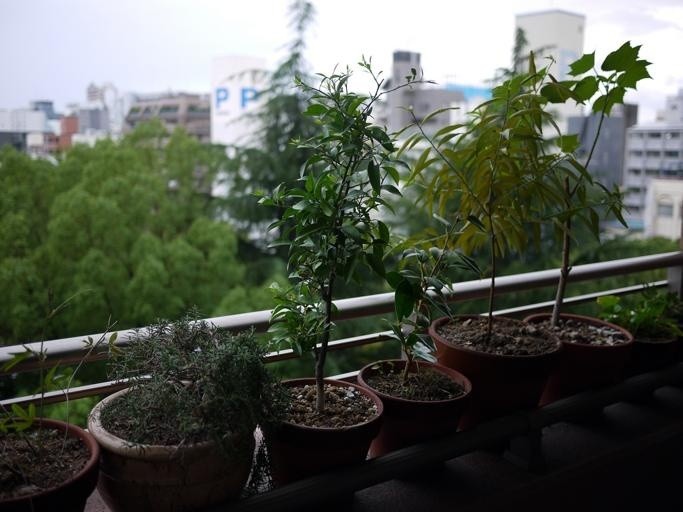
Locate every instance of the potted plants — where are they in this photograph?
[389,52,558,438]
[523,42,653,422]
[2,286,120,507]
[598,275,680,377]
[87,298,299,507]
[246,47,436,482]
[358,214,476,472]
